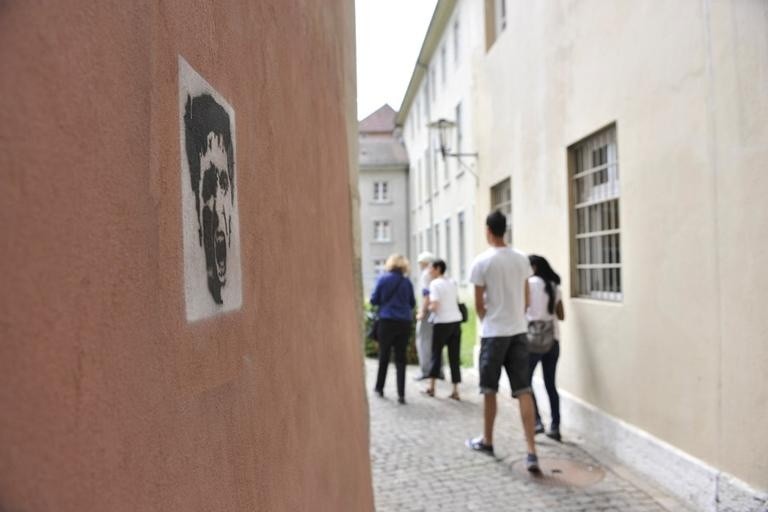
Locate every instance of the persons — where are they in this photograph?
[470,209,538,471]
[423,260,463,401]
[416,253,445,381]
[185,95,234,305]
[370,253,415,403]
[523,253,564,439]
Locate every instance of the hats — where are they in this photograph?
[416,251,435,264]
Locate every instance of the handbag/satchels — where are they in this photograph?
[367,320,379,339]
[459,303,467,323]
[526,320,555,353]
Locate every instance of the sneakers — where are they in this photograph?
[421,388,460,399]
[534,418,560,440]
[465,437,493,454]
[376,391,404,402]
[526,453,539,471]
